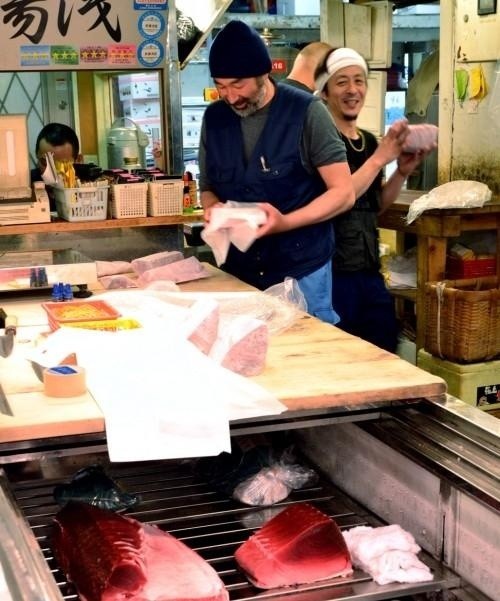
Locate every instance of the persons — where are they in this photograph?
[31,123,99,211]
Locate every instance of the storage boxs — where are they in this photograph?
[415,349,500,412]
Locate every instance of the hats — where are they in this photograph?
[209,20,272,79]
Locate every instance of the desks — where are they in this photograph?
[378,190,500,346]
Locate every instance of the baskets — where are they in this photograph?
[53,179,186,222]
[424,252,500,363]
[40,300,141,331]
